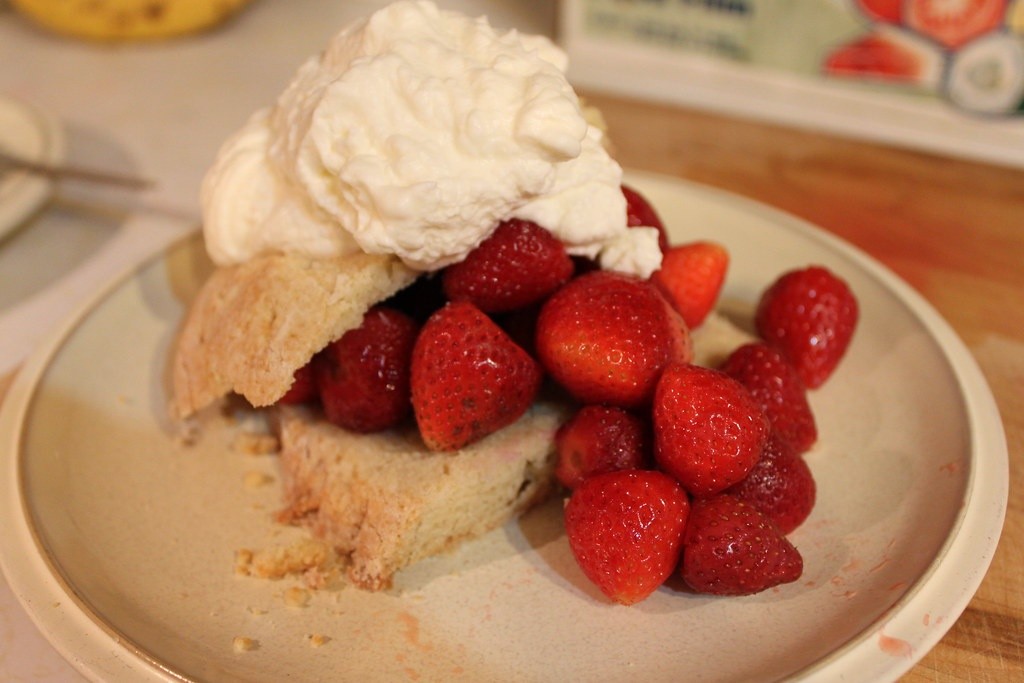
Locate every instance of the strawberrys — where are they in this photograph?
[324,190,858,604]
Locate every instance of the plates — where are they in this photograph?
[1,94,66,239]
[0,171,1010,683]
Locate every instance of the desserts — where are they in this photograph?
[170,0,743,578]
